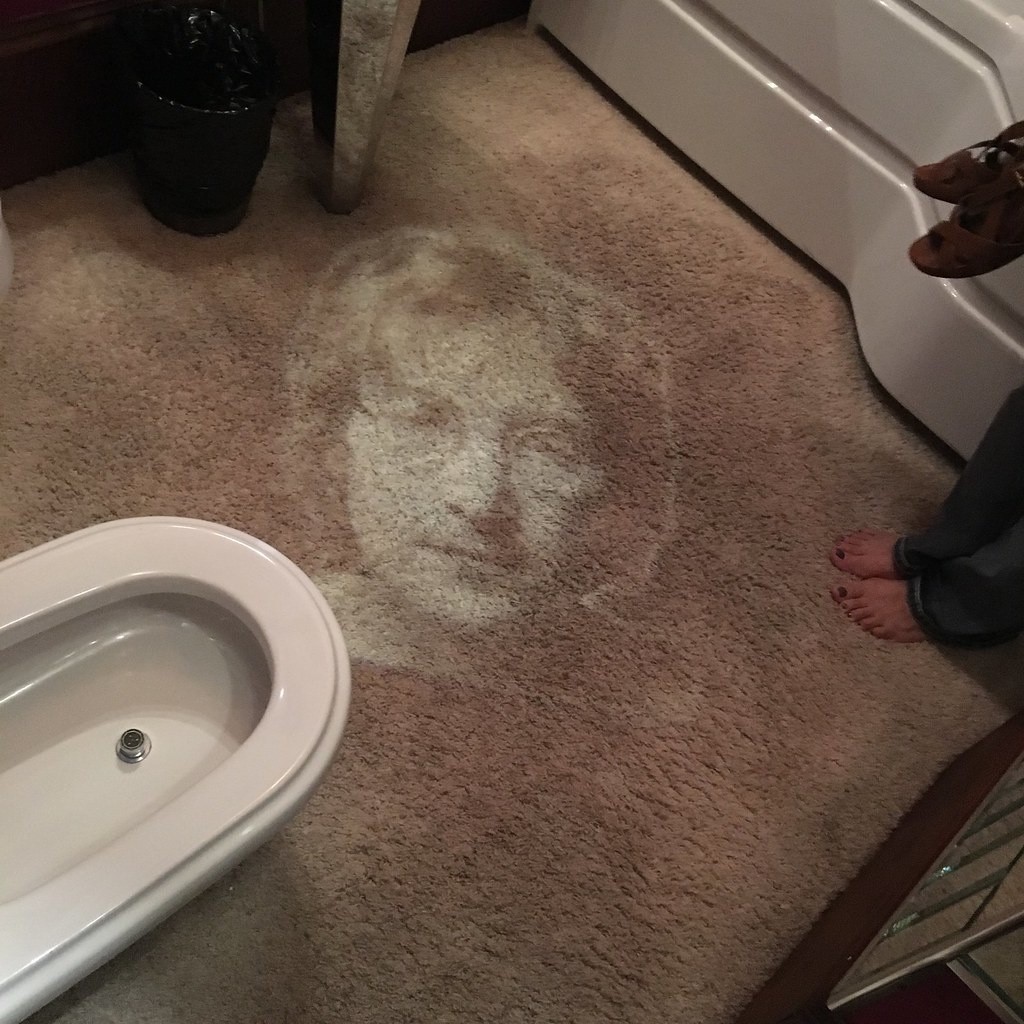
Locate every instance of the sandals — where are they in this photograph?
[908,168,1024,279]
[912,120,1024,205]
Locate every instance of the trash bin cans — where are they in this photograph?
[110,0,291,238]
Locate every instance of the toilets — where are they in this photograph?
[0,514,351,1024]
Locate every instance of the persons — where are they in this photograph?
[288,226,682,689]
[828,386,1024,647]
